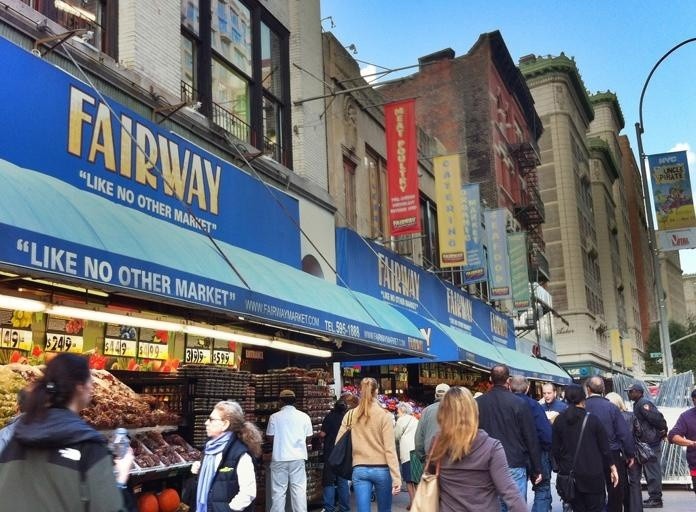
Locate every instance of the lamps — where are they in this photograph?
[331,21,336,28]
[350,43,358,54]
[57,0,96,24]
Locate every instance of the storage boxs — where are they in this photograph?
[108,428,205,481]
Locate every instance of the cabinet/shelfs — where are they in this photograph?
[111,370,183,416]
[260,368,335,512]
[186,366,260,512]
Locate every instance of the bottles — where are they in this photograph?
[107,427,131,475]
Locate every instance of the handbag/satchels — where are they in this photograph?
[633,432,659,464]
[557,412,591,504]
[409,433,442,512]
[325,410,355,479]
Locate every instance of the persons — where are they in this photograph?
[395,400,420,510]
[0,351,136,512]
[605,391,643,512]
[624,384,668,508]
[414,383,451,465]
[0,381,40,455]
[181,400,262,512]
[552,384,619,512]
[425,385,529,512]
[585,376,636,512]
[474,364,543,512]
[540,382,568,424]
[319,393,359,512]
[334,377,403,512]
[265,389,314,512]
[667,389,696,493]
[509,375,552,512]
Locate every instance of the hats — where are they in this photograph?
[624,383,644,393]
[277,390,296,398]
[435,383,451,397]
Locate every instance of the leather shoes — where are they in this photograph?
[643,500,663,509]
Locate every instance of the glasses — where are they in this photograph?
[206,416,222,424]
